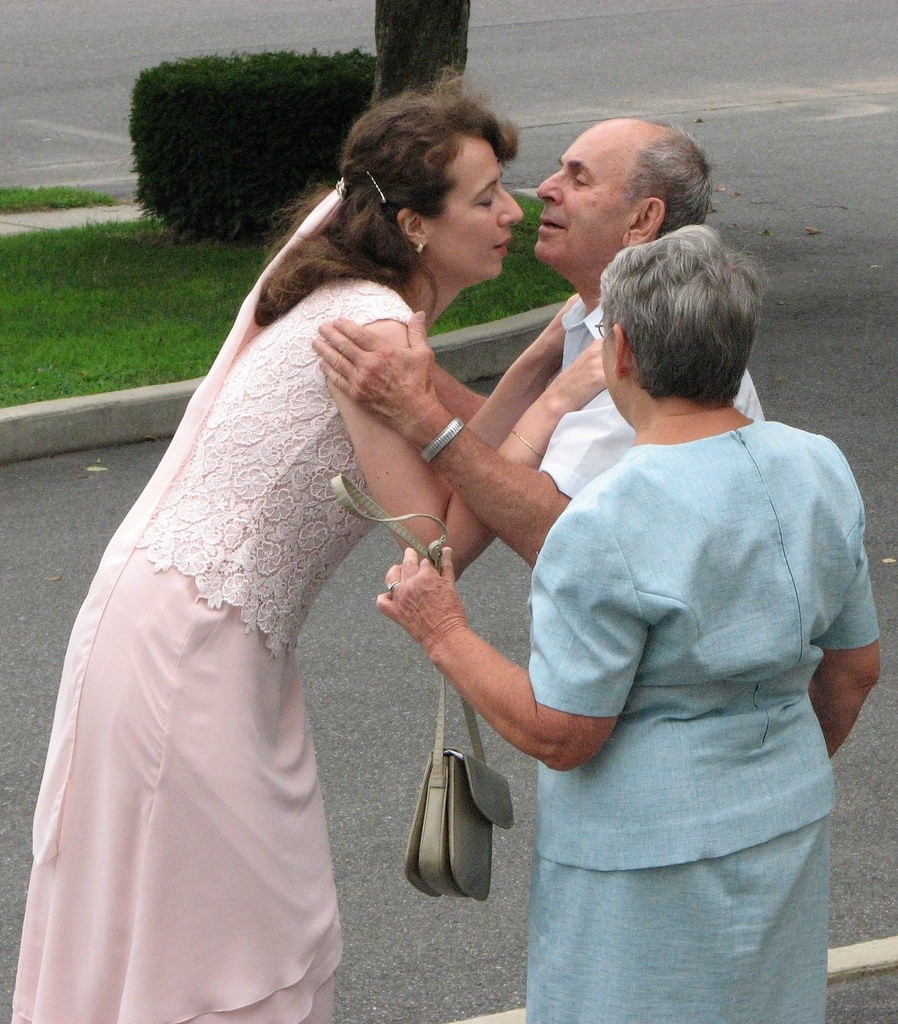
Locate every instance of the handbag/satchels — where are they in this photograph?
[405,746,515,902]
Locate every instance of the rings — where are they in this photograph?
[389,581,400,592]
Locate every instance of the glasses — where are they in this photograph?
[594,318,613,339]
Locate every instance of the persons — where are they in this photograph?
[10,74,607,1024]
[376,225,880,1024]
[312,119,767,567]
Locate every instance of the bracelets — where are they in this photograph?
[512,430,544,459]
[421,417,465,465]
